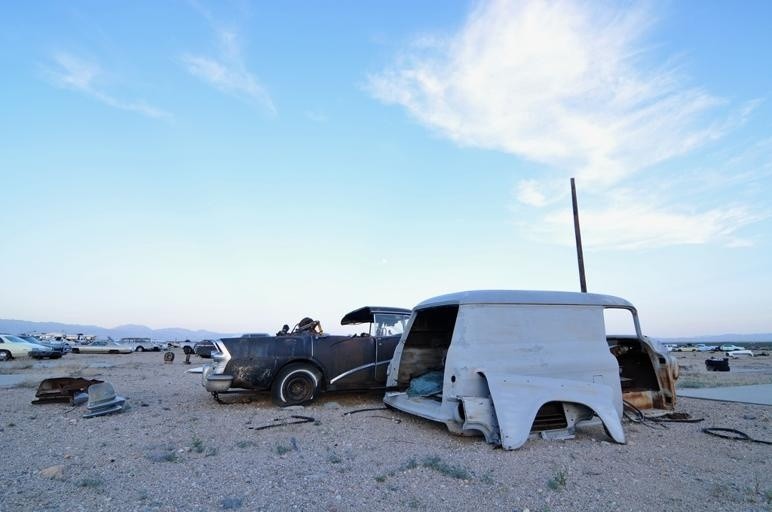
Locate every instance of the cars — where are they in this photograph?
[665,342,747,352]
[205,305,414,405]
[1,332,213,362]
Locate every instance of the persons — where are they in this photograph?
[276,325,292,336]
